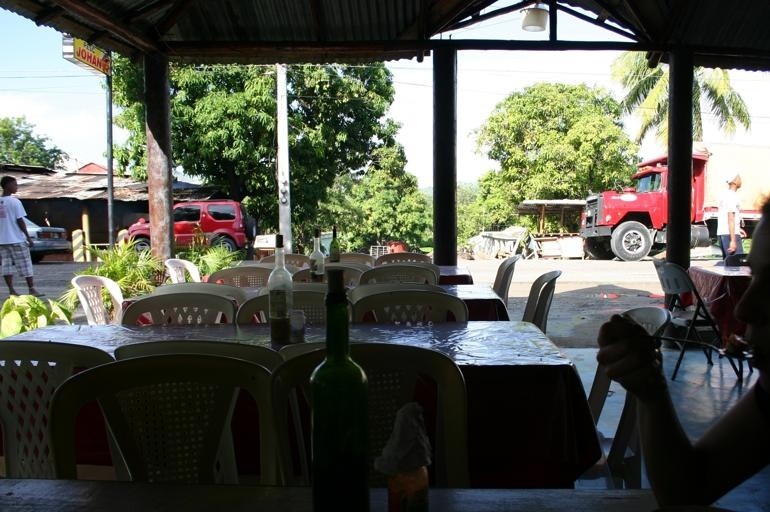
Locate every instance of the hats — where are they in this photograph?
[725,170,738,184]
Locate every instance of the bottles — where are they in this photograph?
[329,227,340,263]
[309,229,325,283]
[386,404,428,511]
[268,235,294,339]
[289,310,306,342]
[310,271,372,512]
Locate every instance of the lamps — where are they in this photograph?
[520,0,549,32]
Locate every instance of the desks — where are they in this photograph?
[1,321,602,487]
[2,486,604,506]
[680,266,754,383]
[122,285,510,320]
[235,261,473,285]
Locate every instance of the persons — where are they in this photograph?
[716,172,746,257]
[598,196,770,512]
[0,176,43,299]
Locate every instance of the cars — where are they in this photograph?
[21,217,71,264]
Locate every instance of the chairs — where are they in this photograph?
[0,339,116,479]
[237,291,351,326]
[493,256,519,305]
[165,259,201,283]
[359,265,437,286]
[352,290,468,324]
[576,306,670,490]
[114,339,280,368]
[121,294,237,326]
[270,342,468,486]
[207,267,272,286]
[153,282,249,307]
[259,283,352,300]
[71,275,123,325]
[374,262,440,283]
[259,253,310,266]
[325,253,375,265]
[654,258,739,381]
[292,266,363,283]
[374,253,431,266]
[352,283,447,303]
[521,271,561,341]
[47,354,273,479]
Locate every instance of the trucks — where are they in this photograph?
[580,69,770,261]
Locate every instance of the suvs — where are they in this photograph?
[124,199,254,253]
